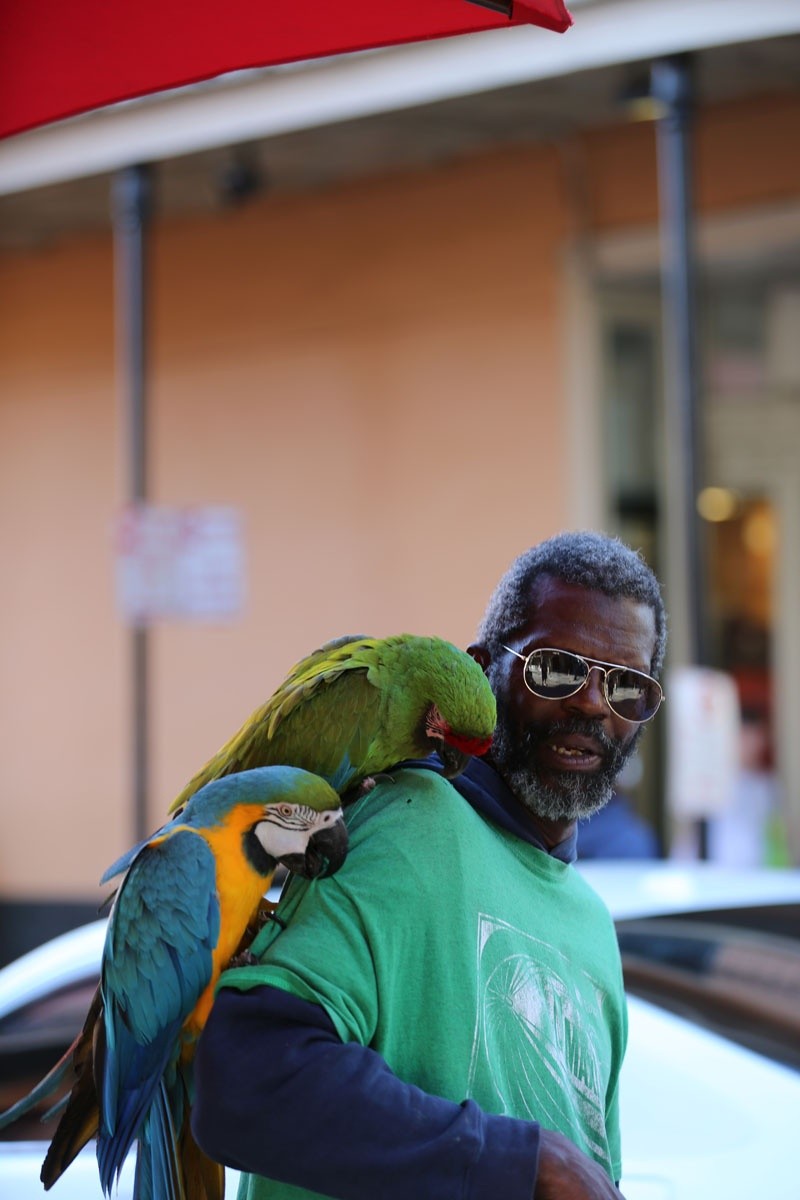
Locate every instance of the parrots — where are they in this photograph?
[167,634,498,803]
[0,762,350,1196]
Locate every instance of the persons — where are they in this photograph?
[608,675,614,702]
[541,658,548,686]
[188,530,669,1200]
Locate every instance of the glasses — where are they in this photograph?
[500,643,666,723]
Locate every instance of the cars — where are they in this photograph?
[1,827,800,1198]
